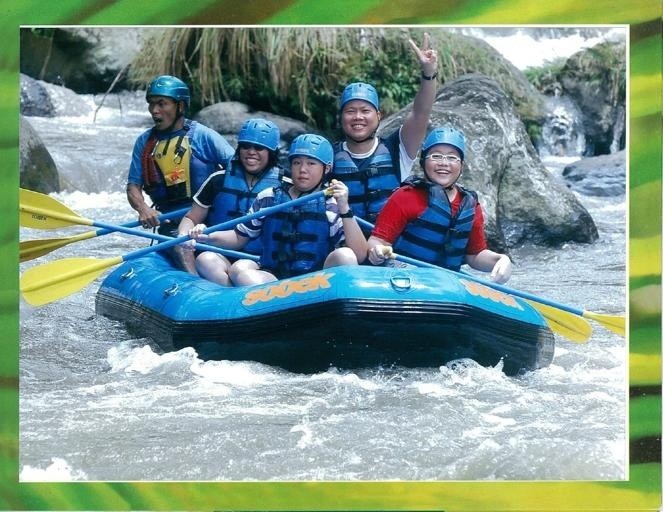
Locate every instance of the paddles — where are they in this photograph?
[18,186,335,307]
[515,296,592,344]
[383,248,626,338]
[19,187,260,262]
[19,207,194,263]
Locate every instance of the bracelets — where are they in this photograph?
[421,70,438,81]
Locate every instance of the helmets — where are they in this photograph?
[288,134,335,171]
[422,127,465,159]
[340,83,378,111]
[238,118,280,150]
[147,74,190,110]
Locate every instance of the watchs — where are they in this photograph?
[339,208,354,218]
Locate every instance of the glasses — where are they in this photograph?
[425,154,461,163]
[241,142,264,151]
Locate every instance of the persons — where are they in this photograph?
[187,134,364,288]
[128,78,237,271]
[178,117,284,286]
[366,127,512,286]
[320,32,439,239]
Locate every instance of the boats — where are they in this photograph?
[93,246,555,379]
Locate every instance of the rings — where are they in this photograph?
[143,224,148,227]
